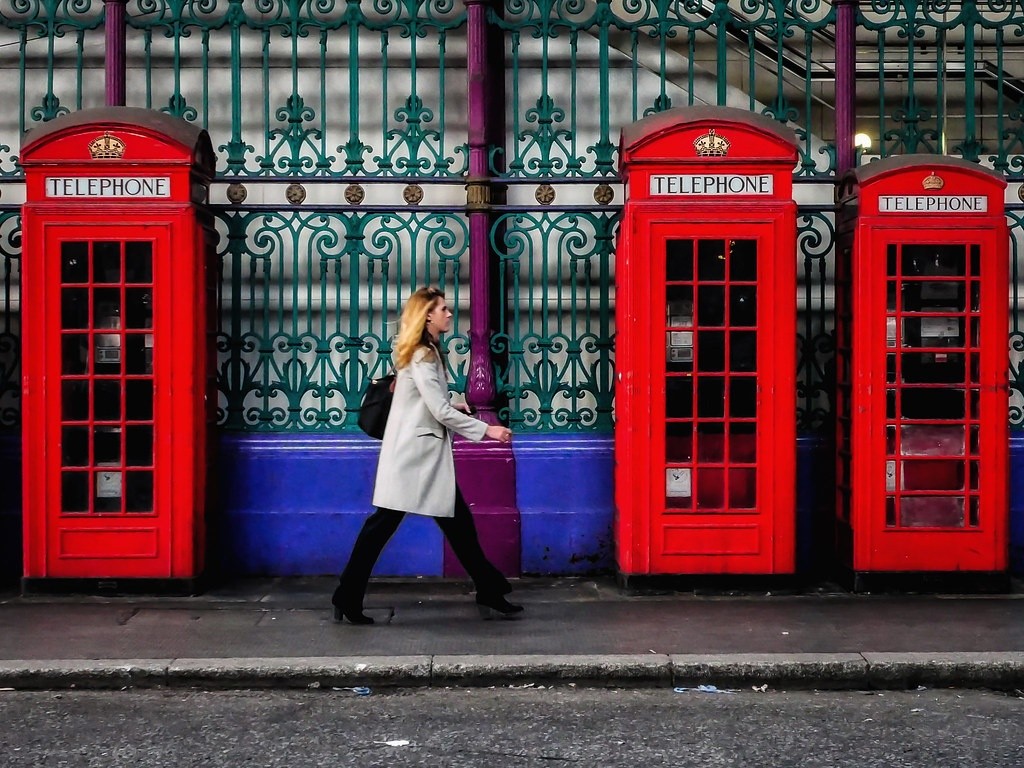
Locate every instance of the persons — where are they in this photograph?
[329,286,525,623]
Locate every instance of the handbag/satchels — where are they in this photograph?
[357,365,397,440]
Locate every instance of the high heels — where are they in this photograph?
[332,589,374,625]
[476,589,524,620]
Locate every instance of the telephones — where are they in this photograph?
[884,306,921,360]
[75,306,127,365]
[663,303,697,364]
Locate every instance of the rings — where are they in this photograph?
[506,434,510,437]
